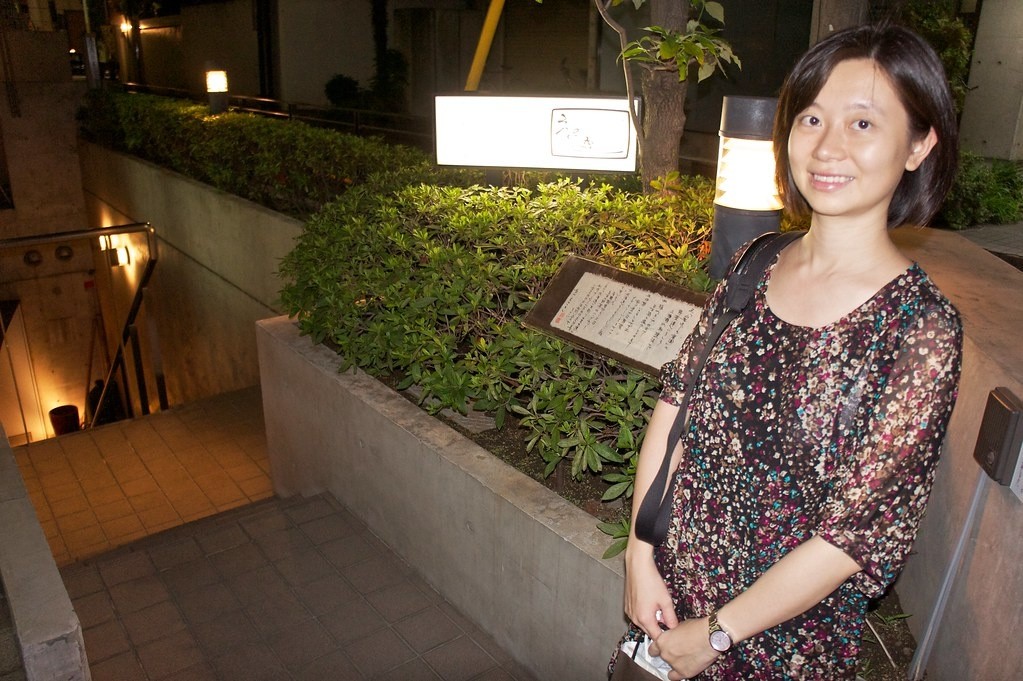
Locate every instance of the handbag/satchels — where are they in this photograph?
[608,623,690,681]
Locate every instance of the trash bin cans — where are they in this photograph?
[49,405,79,436]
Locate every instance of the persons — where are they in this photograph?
[608,22,963,681]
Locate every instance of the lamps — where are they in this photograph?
[204,70,230,115]
[101,245,130,267]
[706,92,788,284]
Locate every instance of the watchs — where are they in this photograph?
[708,610,735,655]
